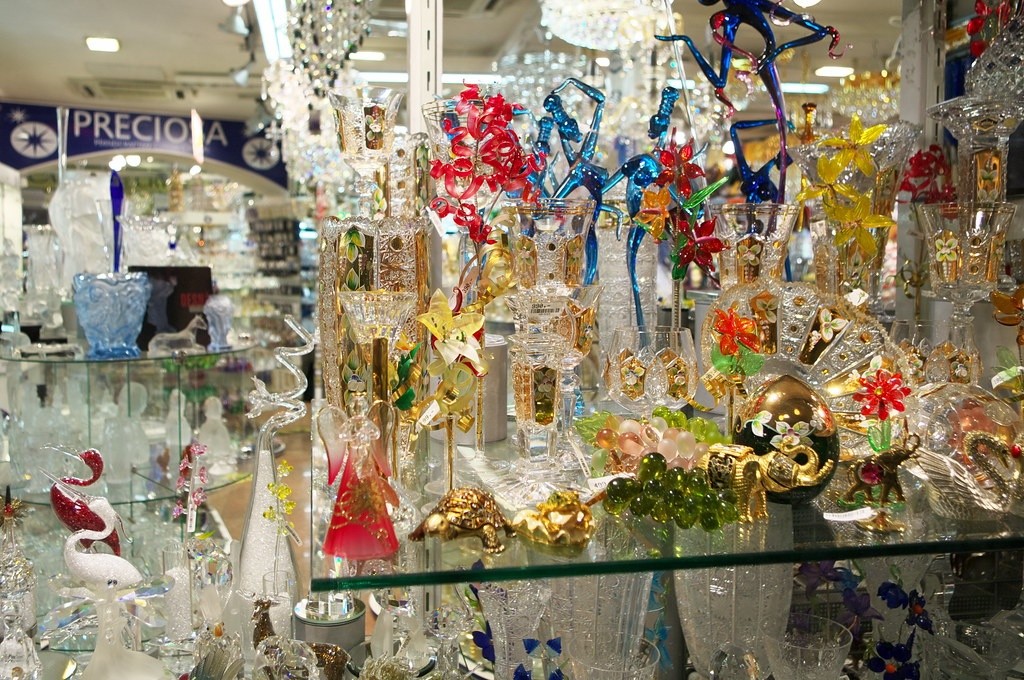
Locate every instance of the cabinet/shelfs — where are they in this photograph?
[0,0,1024,680]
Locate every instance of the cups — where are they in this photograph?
[761,615,853,680]
[73,274,150,357]
[567,632,660,679]
